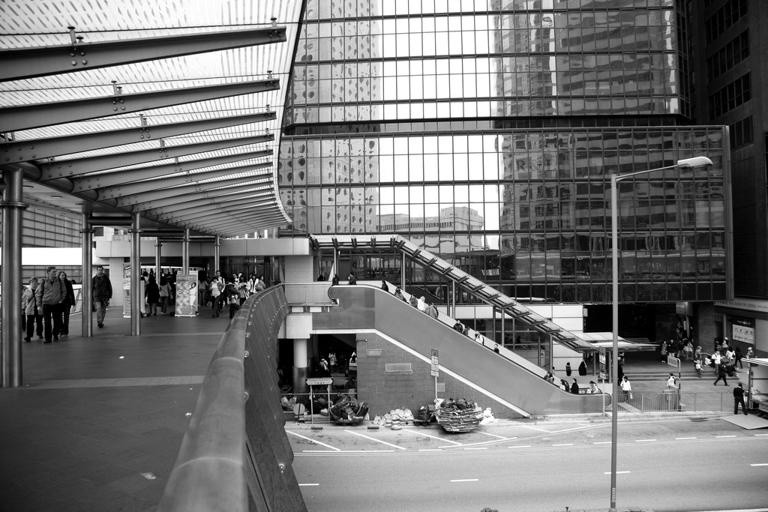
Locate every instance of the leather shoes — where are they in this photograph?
[23,336,58,344]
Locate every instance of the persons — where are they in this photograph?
[566,363,571,376]
[320,350,357,381]
[618,363,624,385]
[561,380,569,392]
[579,362,586,375]
[571,379,578,394]
[91,265,112,328]
[19,267,75,344]
[590,381,599,393]
[348,273,356,285]
[621,376,631,400]
[198,270,266,319]
[333,274,339,285]
[140,267,177,316]
[661,338,755,387]
[733,383,748,415]
[382,279,499,354]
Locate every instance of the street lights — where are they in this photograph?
[609,153,716,511]
